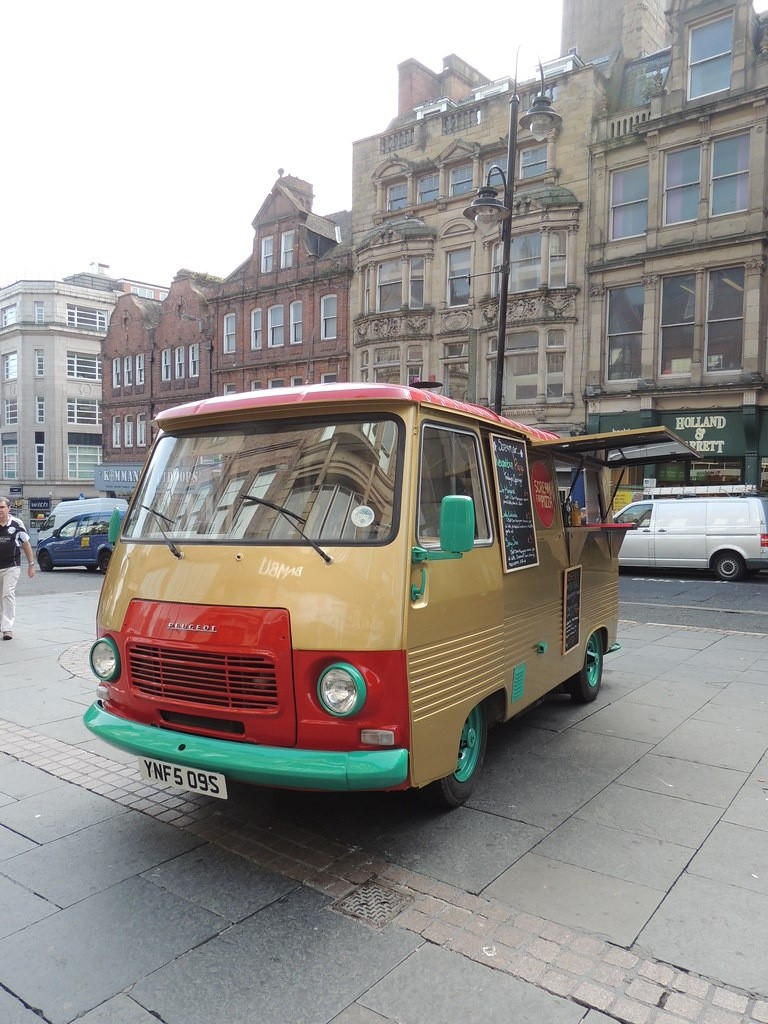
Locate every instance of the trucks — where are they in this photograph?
[78,380,704,812]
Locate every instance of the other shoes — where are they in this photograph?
[3,631,12,640]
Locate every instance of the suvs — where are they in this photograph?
[36,511,127,575]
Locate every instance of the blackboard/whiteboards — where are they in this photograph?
[489,433,540,574]
[563,564,582,655]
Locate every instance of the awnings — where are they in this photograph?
[532,425,704,519]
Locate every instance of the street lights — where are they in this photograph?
[460,44,563,416]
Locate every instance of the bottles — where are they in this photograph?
[563,498,572,527]
[572,501,581,526]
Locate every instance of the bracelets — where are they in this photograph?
[29,562,34,565]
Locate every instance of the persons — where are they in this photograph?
[0,497,35,640]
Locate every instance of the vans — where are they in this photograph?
[36,496,129,544]
[611,482,768,583]
